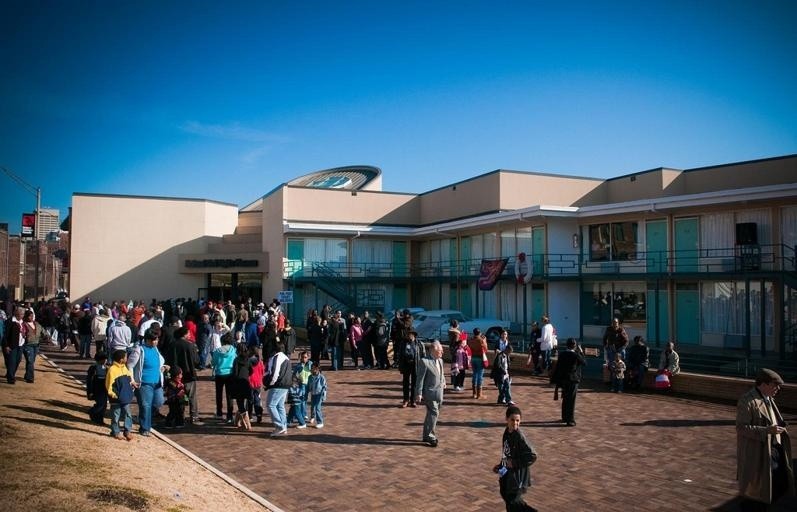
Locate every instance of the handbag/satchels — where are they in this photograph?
[499,470,522,507]
[481,347,489,368]
[655,368,671,388]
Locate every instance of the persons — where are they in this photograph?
[36,297,329,441]
[493,406,540,512]
[591,238,601,250]
[550,336,589,427]
[592,289,646,322]
[0,296,43,385]
[735,363,796,512]
[601,238,610,249]
[601,318,651,393]
[654,341,681,393]
[527,314,557,377]
[398,319,516,407]
[308,304,414,372]
[416,340,446,446]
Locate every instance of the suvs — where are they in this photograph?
[55,288,68,299]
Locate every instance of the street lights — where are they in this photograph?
[43,255,55,300]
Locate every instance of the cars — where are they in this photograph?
[380,307,510,344]
[588,297,647,326]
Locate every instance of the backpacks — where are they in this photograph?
[375,322,387,338]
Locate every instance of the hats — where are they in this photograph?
[753,368,785,385]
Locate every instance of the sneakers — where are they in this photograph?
[139,427,150,437]
[88,408,200,428]
[123,432,134,441]
[401,399,417,408]
[7,373,34,384]
[327,362,399,371]
[422,433,438,447]
[449,385,465,393]
[110,431,126,441]
[270,415,324,437]
[531,368,553,377]
[496,400,519,407]
[214,415,234,426]
[559,419,576,427]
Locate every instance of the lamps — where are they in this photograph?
[572,234,581,248]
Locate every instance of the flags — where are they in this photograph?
[477,257,509,291]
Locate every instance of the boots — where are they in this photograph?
[472,385,488,400]
[235,405,263,432]
